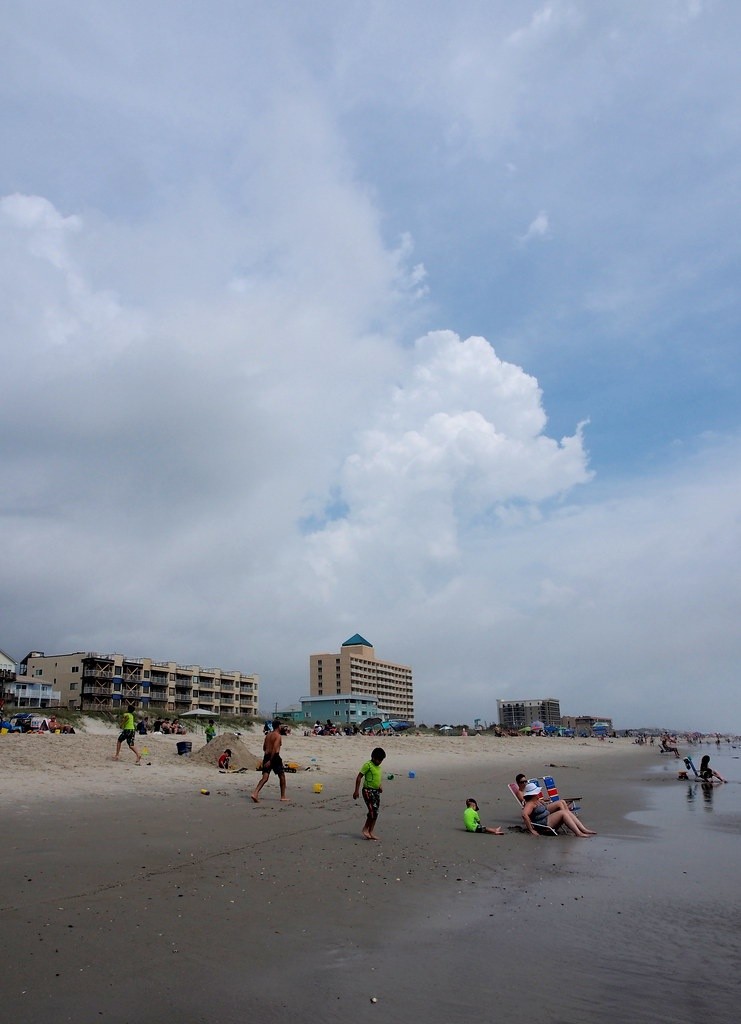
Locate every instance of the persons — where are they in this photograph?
[204,721,217,745]
[153,716,187,735]
[314,719,404,736]
[353,747,387,840]
[515,773,598,838]
[47,715,75,734]
[263,721,293,735]
[699,754,728,784]
[492,726,741,759]
[112,705,141,763]
[137,716,149,735]
[218,749,231,769]
[251,720,291,803]
[464,798,505,835]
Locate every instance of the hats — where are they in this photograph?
[466,798,479,811]
[523,783,543,796]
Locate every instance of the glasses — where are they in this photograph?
[519,779,528,784]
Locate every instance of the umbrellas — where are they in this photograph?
[591,722,610,735]
[517,720,575,734]
[439,726,453,735]
[359,717,410,729]
[181,709,220,735]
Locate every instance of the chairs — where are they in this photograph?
[508,776,583,836]
[658,744,675,757]
[684,755,715,783]
